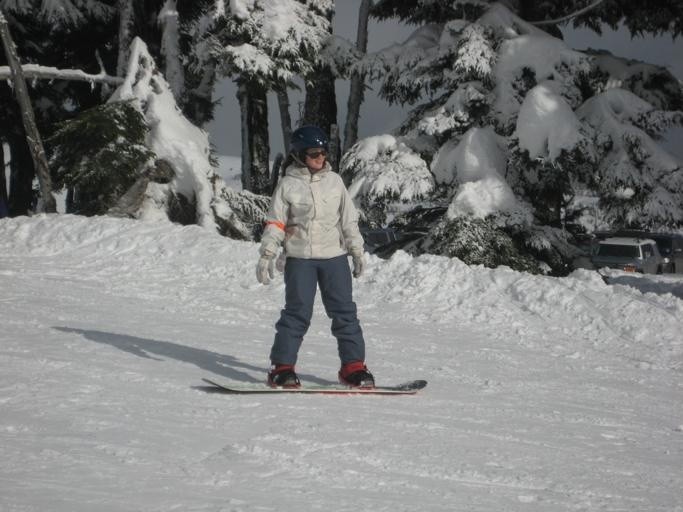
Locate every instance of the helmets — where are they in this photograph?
[289,125,328,152]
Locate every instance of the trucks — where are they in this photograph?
[591,231,683,280]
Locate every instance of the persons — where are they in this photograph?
[253,124,375,390]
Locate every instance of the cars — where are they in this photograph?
[357,223,400,251]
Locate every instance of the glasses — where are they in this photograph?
[305,148,327,159]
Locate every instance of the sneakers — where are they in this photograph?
[271,366,296,386]
[341,364,374,386]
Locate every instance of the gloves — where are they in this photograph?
[350,250,363,278]
[256,256,274,285]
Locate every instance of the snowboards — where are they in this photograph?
[202,377,428,395]
